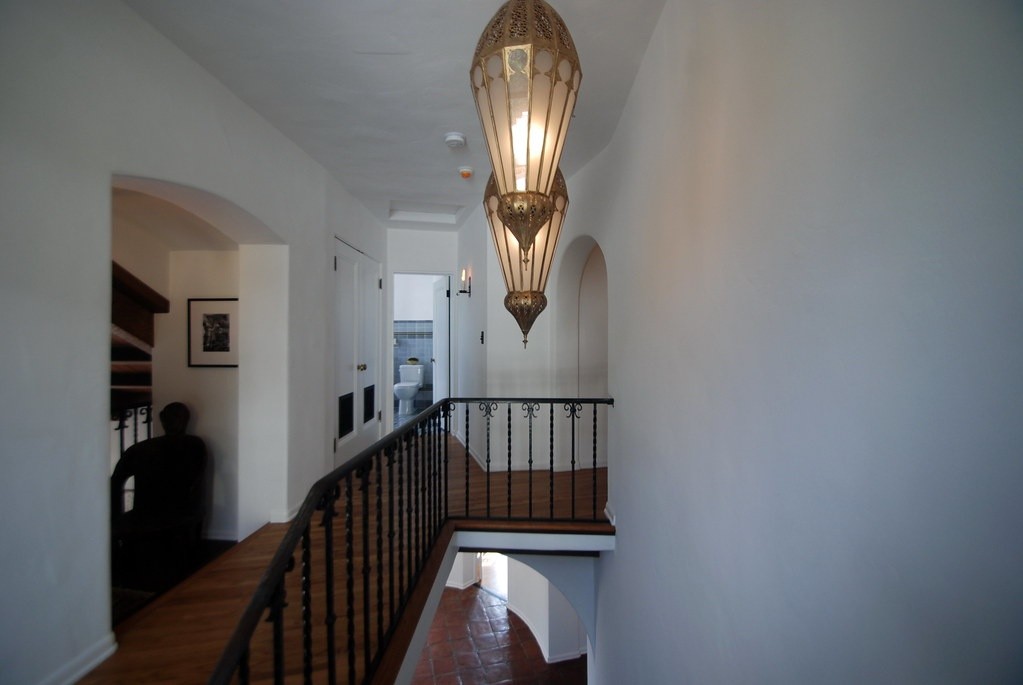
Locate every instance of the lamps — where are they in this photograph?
[468,1,583,272]
[459,268,471,298]
[482,164,567,348]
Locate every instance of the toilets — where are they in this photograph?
[394,365,423,415]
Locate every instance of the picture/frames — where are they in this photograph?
[187,296,239,367]
